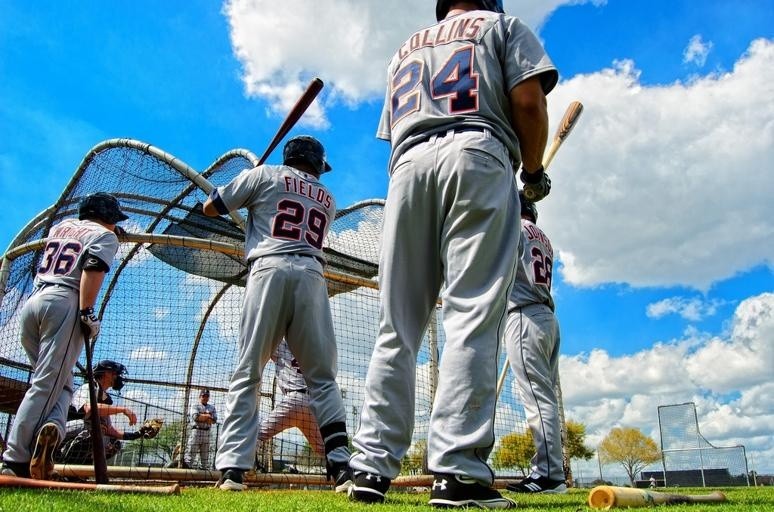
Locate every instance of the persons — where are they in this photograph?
[0,191,127,478]
[48,355,163,484]
[647,473,656,488]
[499,188,571,493]
[184,386,218,468]
[247,334,334,471]
[345,0,559,510]
[198,136,363,492]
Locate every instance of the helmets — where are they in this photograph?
[92,358,129,392]
[283,133,332,172]
[519,190,538,225]
[79,191,128,223]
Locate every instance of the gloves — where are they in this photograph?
[78,307,101,338]
[521,165,551,202]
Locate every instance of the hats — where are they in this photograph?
[199,388,210,397]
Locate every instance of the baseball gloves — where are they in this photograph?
[138,418,163,439]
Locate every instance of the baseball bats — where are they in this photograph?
[79,321,109,483]
[0,475,181,497]
[256,78,324,167]
[523,102,583,199]
[588,484,726,510]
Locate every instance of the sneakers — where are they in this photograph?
[506,471,567,495]
[428,472,517,510]
[2,423,60,479]
[218,468,244,492]
[333,466,391,502]
